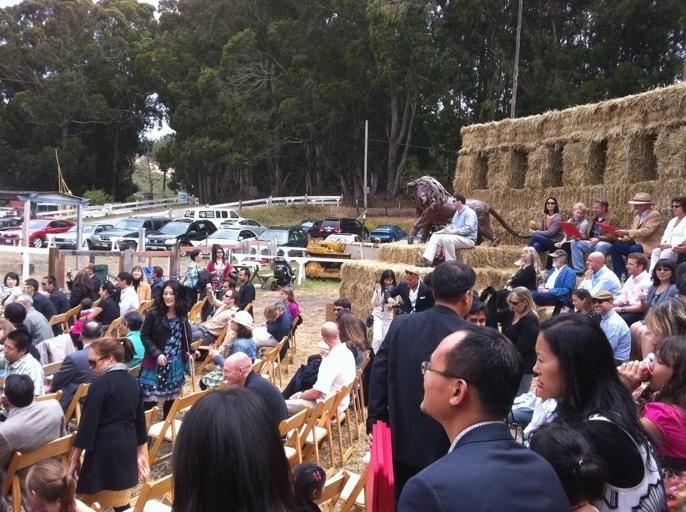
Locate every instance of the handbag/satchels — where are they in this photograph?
[366,314,373,327]
[432,244,446,268]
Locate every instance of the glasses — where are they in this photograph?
[545,202,555,205]
[89,354,110,370]
[333,308,342,312]
[404,269,418,277]
[593,299,608,304]
[419,361,466,384]
[508,300,525,306]
[223,293,233,298]
[670,205,681,208]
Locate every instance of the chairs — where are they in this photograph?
[1,297,369,511]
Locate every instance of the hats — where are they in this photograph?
[628,192,655,206]
[592,292,612,300]
[549,249,567,257]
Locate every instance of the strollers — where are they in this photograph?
[269,256,298,291]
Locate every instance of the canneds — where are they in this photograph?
[640,365,651,382]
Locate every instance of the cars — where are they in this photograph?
[0,201,130,251]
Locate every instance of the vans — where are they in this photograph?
[182,207,244,228]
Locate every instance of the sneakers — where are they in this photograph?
[415,258,433,268]
[514,257,526,266]
